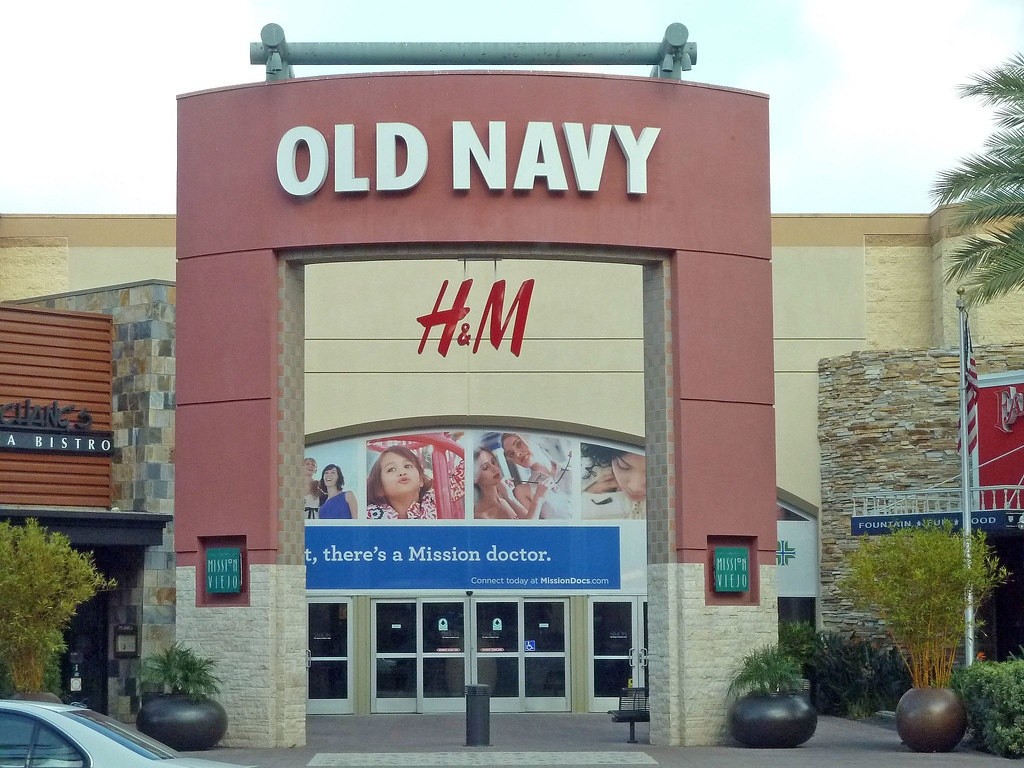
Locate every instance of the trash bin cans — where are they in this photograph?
[463,684,493,747]
[141,680,165,706]
[783,678,811,704]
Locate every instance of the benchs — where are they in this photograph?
[607,687,651,744]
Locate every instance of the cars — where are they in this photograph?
[1,699,257,767]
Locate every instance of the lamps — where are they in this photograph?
[271,52,283,71]
[662,53,675,73]
[266,55,277,76]
[679,52,693,71]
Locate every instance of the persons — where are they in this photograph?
[367,445,466,520]
[501,433,572,519]
[304,457,358,519]
[474,446,548,519]
[581,443,646,501]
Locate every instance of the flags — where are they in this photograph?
[958,321,978,453]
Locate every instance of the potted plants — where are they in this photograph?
[0,517,119,704]
[835,518,1014,753]
[136,639,228,751]
[724,641,817,749]
